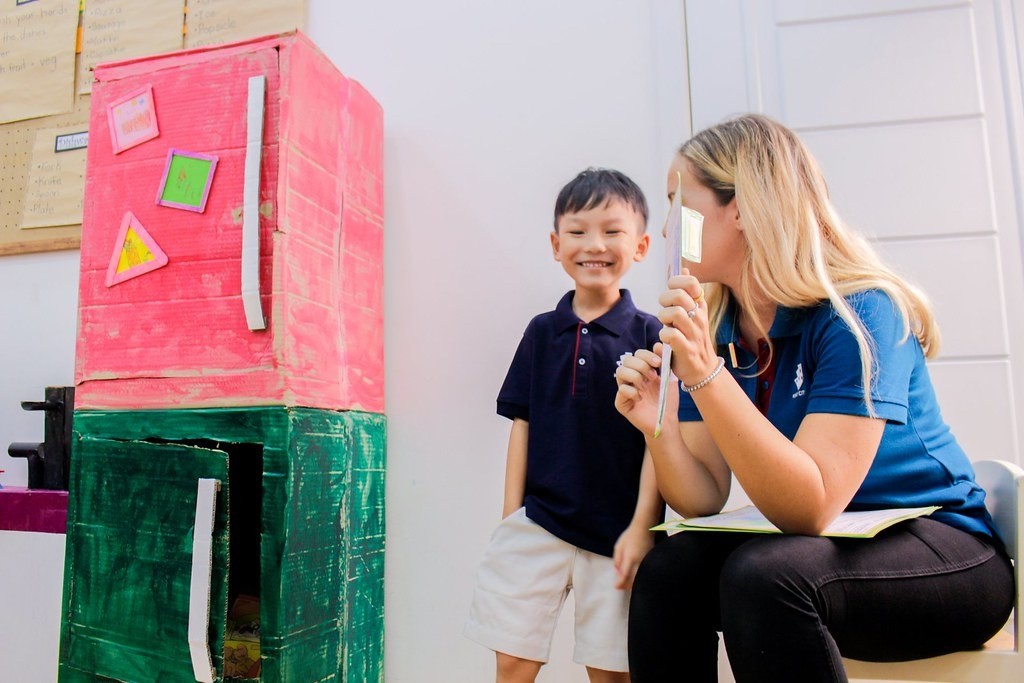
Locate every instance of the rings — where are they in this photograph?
[694,290,704,302]
[688,303,699,317]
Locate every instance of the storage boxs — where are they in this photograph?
[0,486,68,683]
[55,27,386,683]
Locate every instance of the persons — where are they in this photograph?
[615,114,1015,683]
[468,168,665,683]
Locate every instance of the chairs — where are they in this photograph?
[839,459,1024,683]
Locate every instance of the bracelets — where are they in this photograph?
[681,357,726,391]
